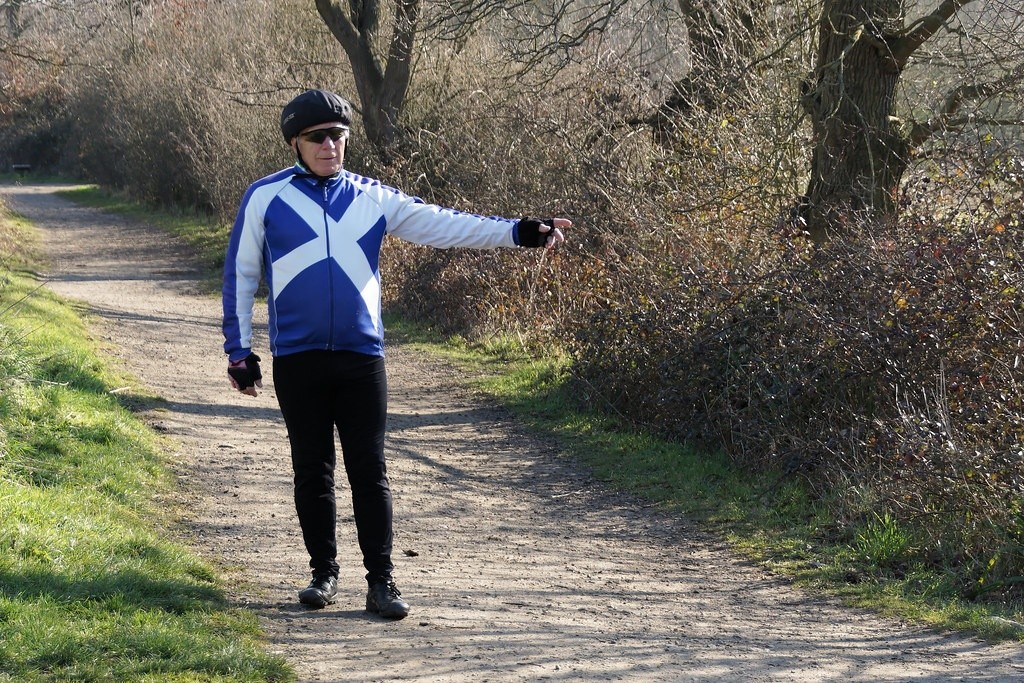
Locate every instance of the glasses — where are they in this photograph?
[301,127,345,144]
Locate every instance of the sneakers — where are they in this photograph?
[299,570,338,605]
[366,580,409,616]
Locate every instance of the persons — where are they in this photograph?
[221,91,572,619]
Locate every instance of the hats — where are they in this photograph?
[281,90,353,146]
